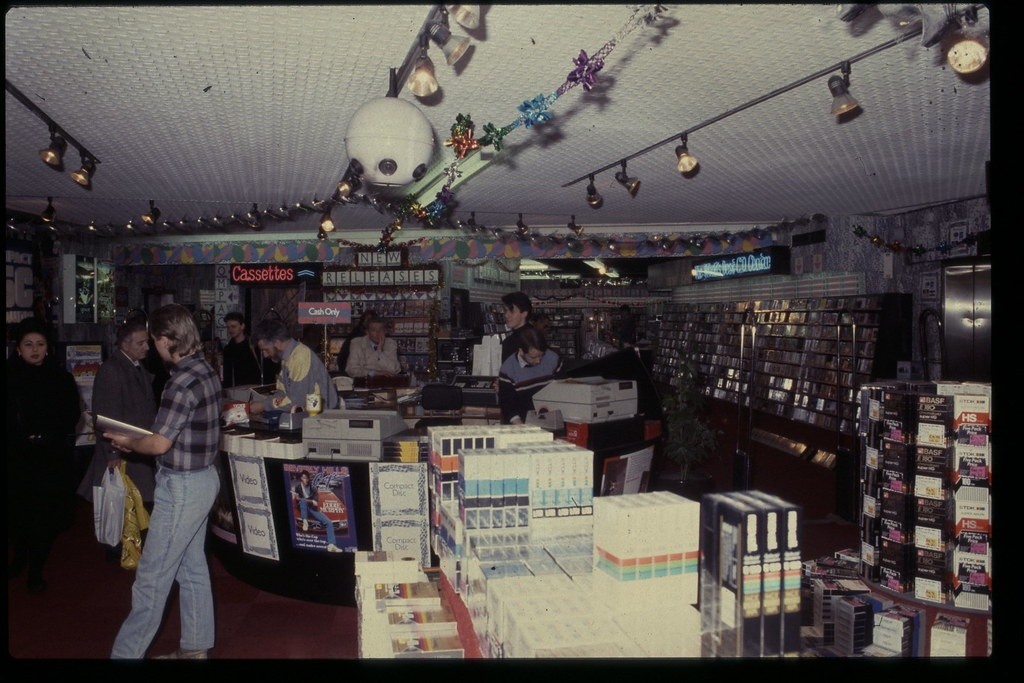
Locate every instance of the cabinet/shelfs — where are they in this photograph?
[323,281,436,389]
[489,327,578,362]
[438,335,477,363]
[529,305,650,345]
[651,290,911,466]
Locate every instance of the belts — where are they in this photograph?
[156,462,214,474]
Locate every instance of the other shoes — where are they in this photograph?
[327,544,343,553]
[8,557,27,577]
[24,575,45,592]
[302,518,308,532]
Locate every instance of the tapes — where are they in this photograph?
[291,405,304,413]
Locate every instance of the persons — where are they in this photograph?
[223,312,271,387]
[0,317,85,597]
[295,470,343,552]
[502,292,538,360]
[338,309,378,376]
[251,309,341,412]
[92,321,158,498]
[498,328,564,425]
[346,318,401,377]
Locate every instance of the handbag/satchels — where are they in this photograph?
[92,466,126,547]
[120,459,142,571]
[125,475,150,531]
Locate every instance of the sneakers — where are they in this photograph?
[152,650,207,660]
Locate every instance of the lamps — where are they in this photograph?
[586,172,601,206]
[828,62,863,117]
[336,176,365,197]
[248,203,259,218]
[568,214,583,235]
[43,196,56,223]
[407,5,485,100]
[615,159,639,194]
[671,138,697,174]
[943,26,989,74]
[38,126,66,166]
[141,199,162,224]
[468,212,487,234]
[71,158,95,186]
[318,214,338,240]
[517,214,528,235]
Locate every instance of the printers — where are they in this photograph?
[533,378,638,425]
[302,409,408,461]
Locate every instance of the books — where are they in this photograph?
[95,414,154,437]
[66,289,992,658]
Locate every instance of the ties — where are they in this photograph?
[374,346,377,352]
[136,366,141,372]
